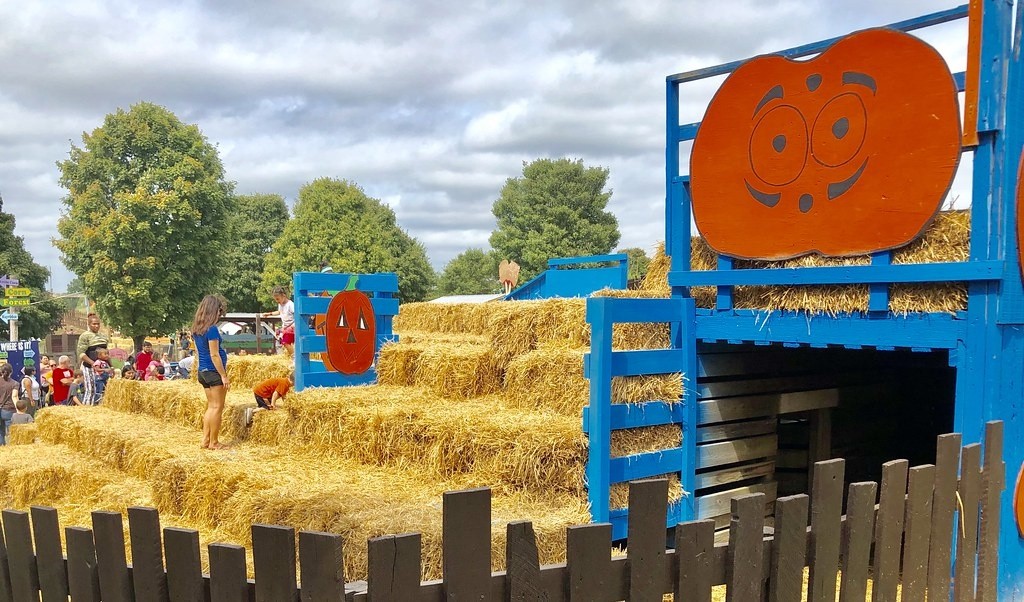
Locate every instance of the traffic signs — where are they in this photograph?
[0,274,19,288]
[0,311,19,324]
[4,287,31,297]
[0,299,30,307]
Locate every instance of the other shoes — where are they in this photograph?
[209,443,232,450]
[201,445,208,448]
[244,407,253,427]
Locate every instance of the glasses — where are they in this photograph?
[50,364,57,367]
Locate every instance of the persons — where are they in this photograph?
[244,368,295,427]
[193,296,231,447]
[0,313,195,448]
[253,262,333,369]
